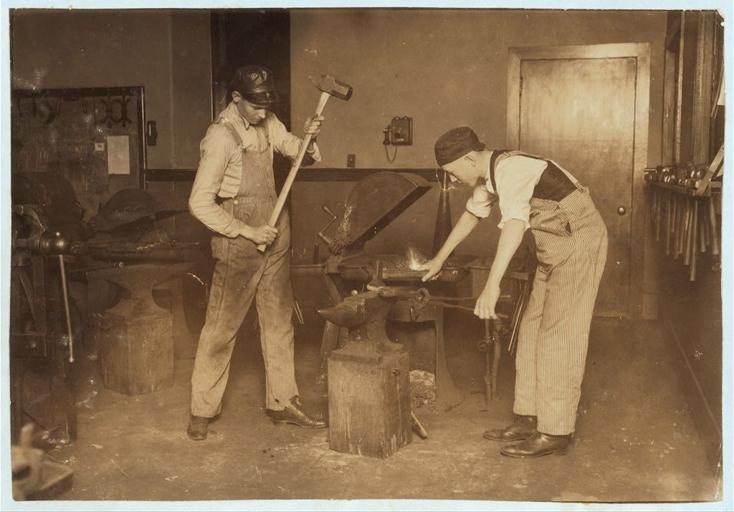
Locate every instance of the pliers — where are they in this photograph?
[380,289,514,319]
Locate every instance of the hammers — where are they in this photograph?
[257,75,353,251]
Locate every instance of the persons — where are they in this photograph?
[404,124,609,461]
[185,61,328,438]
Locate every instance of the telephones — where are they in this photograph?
[383,116,414,146]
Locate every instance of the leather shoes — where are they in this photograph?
[266,395,327,429]
[187,415,208,441]
[482,415,537,442]
[500,431,571,457]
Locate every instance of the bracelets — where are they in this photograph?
[305,148,315,154]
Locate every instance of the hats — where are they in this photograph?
[434,127,485,166]
[230,64,276,104]
